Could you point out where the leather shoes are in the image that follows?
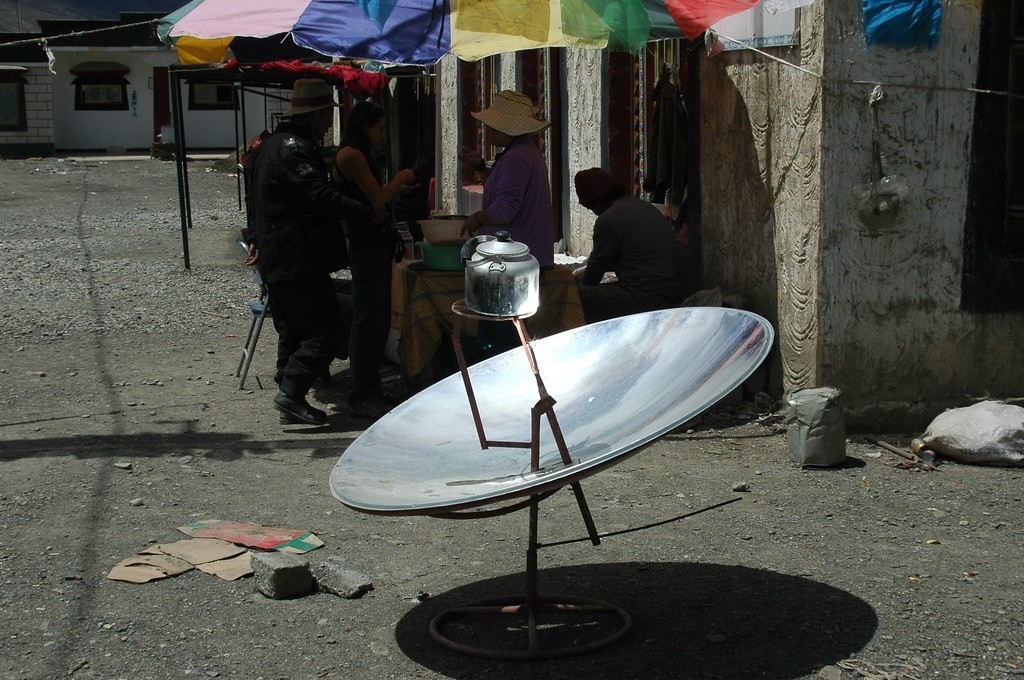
[272,390,328,425]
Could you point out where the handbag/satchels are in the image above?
[395,233,405,263]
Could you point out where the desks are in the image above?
[385,259,586,392]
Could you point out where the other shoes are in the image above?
[349,392,393,417]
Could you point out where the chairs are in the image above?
[234,229,272,391]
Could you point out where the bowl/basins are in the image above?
[417,220,479,242]
[416,242,465,271]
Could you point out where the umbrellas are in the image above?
[157,0,757,216]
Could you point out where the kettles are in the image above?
[462,231,540,320]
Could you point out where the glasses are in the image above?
[366,100,382,121]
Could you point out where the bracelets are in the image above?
[474,211,483,226]
[474,160,485,170]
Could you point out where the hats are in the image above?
[288,78,339,114]
[471,90,550,136]
[575,168,619,205]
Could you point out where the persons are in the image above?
[245,79,364,424]
[575,168,691,321]
[460,89,554,362]
[333,101,415,413]
